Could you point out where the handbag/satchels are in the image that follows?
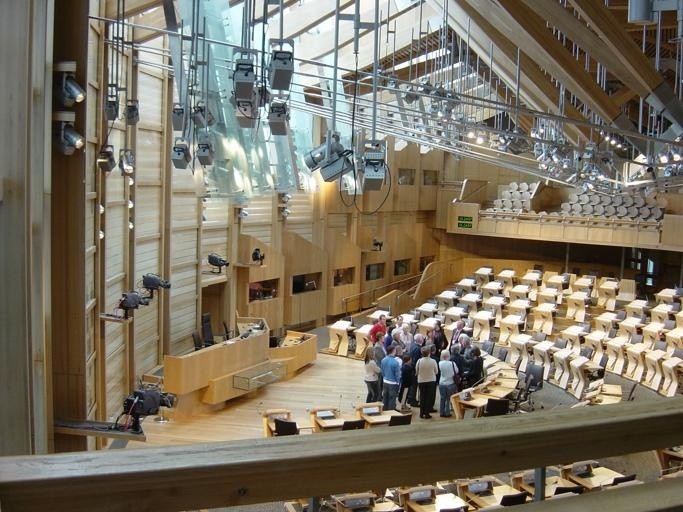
[453,373,462,385]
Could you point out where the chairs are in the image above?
[188,311,232,352]
[327,263,683,418]
[263,402,681,511]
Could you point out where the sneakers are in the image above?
[400,399,452,417]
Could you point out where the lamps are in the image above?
[529,84,683,195]
[227,0,300,137]
[50,58,143,179]
[303,2,397,217]
[386,0,528,158]
[167,0,221,173]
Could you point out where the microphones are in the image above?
[351,396,360,409]
[256,402,267,417]
[470,353,503,394]
[338,394,342,412]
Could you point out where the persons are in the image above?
[364,314,485,419]
